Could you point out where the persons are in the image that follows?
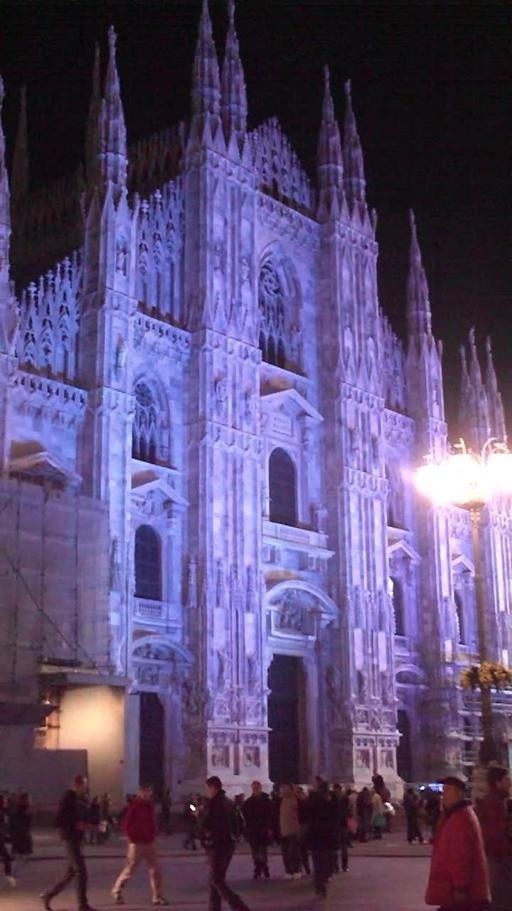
[199,776,251,911]
[242,769,395,899]
[40,772,101,911]
[0,789,42,887]
[235,793,245,842]
[84,788,172,847]
[424,762,512,911]
[181,793,211,853]
[110,775,169,905]
[403,786,444,845]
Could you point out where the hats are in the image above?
[436,776,469,791]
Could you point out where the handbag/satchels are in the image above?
[346,817,358,833]
[99,820,107,833]
[372,816,386,828]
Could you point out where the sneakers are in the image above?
[252,867,350,880]
[78,904,97,911]
[152,896,169,905]
[110,892,124,903]
[40,893,52,911]
